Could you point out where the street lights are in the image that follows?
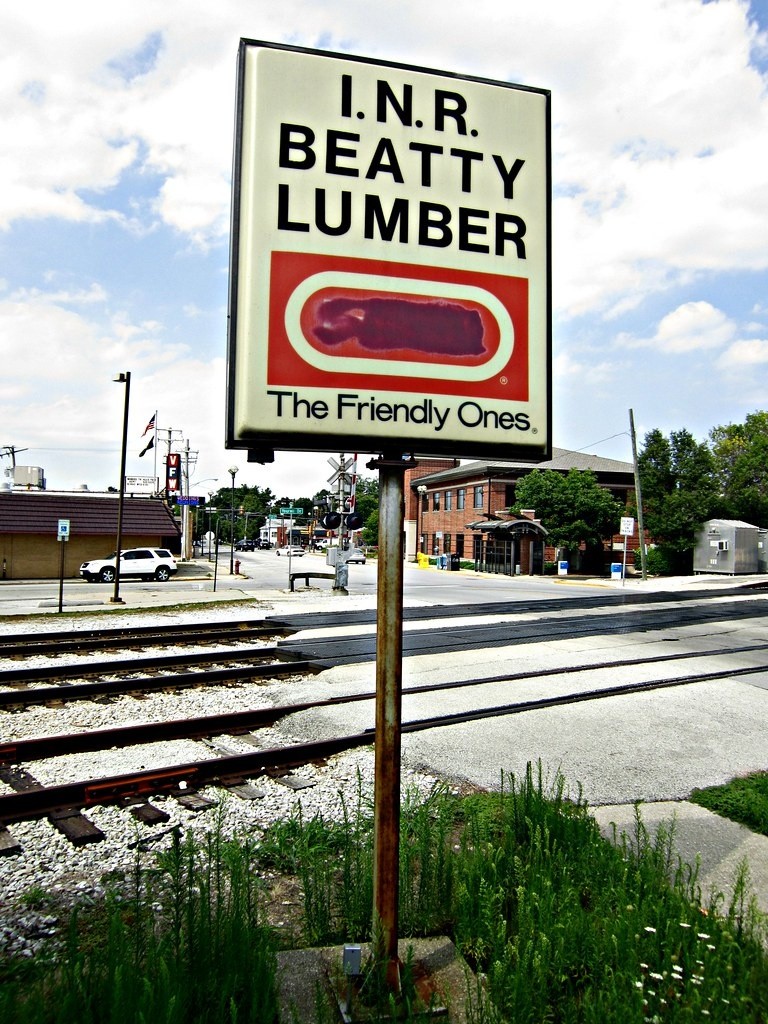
[227,466,238,575]
[112,369,132,600]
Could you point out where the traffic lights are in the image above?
[326,512,343,529]
[346,512,365,532]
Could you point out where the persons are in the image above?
[127,552,136,559]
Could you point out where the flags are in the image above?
[139,436,154,457]
[140,415,155,437]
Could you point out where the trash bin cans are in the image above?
[417,551,430,569]
[447,552,461,571]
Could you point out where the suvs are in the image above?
[78,545,178,582]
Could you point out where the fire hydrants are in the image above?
[235,559,241,575]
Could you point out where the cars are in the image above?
[276,545,306,558]
[345,548,366,565]
[314,537,344,550]
[233,538,273,551]
